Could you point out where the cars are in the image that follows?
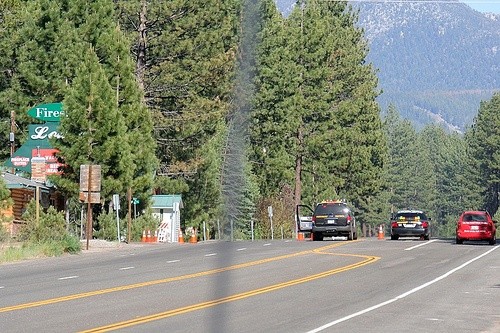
[453,211,498,244]
[389,209,431,241]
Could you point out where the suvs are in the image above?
[297,202,357,241]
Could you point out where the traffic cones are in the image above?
[141,231,157,243]
[376,224,384,239]
[178,231,184,244]
[189,231,198,244]
[297,233,304,239]
